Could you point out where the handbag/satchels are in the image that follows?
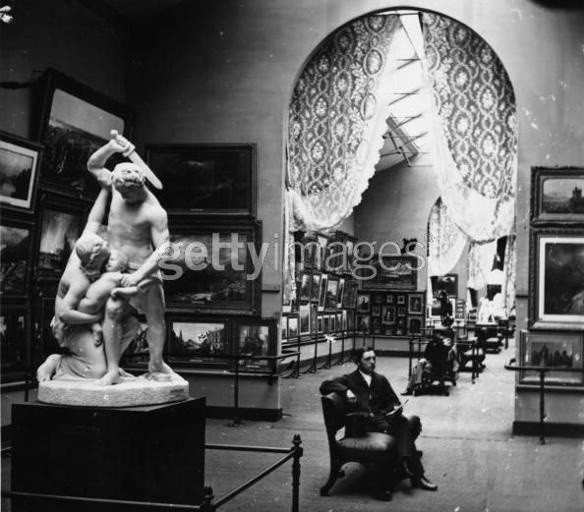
[346,413,370,438]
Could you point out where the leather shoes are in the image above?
[412,476,437,490]
[398,461,414,477]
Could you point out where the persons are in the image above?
[36,167,139,382]
[87,134,175,386]
[321,346,438,491]
[77,251,139,346]
[401,329,453,395]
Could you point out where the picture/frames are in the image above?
[0,67,277,394]
[282,230,426,348]
[528,229,584,329]
[529,166,584,227]
[519,330,584,386]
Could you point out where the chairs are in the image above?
[457,336,485,376]
[423,350,456,395]
[320,394,398,501]
[477,327,503,353]
